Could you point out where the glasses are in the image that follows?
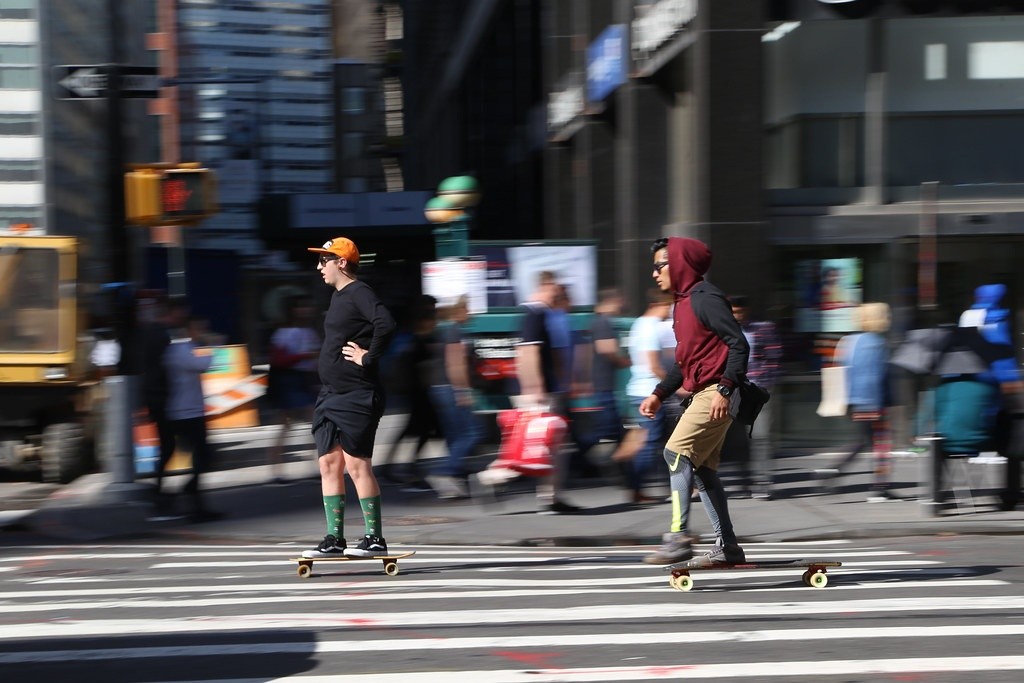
[318,255,341,265]
[650,261,669,272]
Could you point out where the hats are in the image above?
[307,237,360,264]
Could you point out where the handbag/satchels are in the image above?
[491,410,567,474]
[728,381,770,425]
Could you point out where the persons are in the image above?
[147,271,1024,525]
[638,237,750,567]
[300,236,395,559]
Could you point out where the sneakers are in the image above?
[688,544,746,565]
[344,533,388,557]
[644,537,694,563]
[302,534,347,557]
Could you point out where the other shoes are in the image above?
[538,502,579,515]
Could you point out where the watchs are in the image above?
[717,385,733,397]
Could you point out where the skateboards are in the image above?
[663,561,842,592]
[289,550,417,578]
